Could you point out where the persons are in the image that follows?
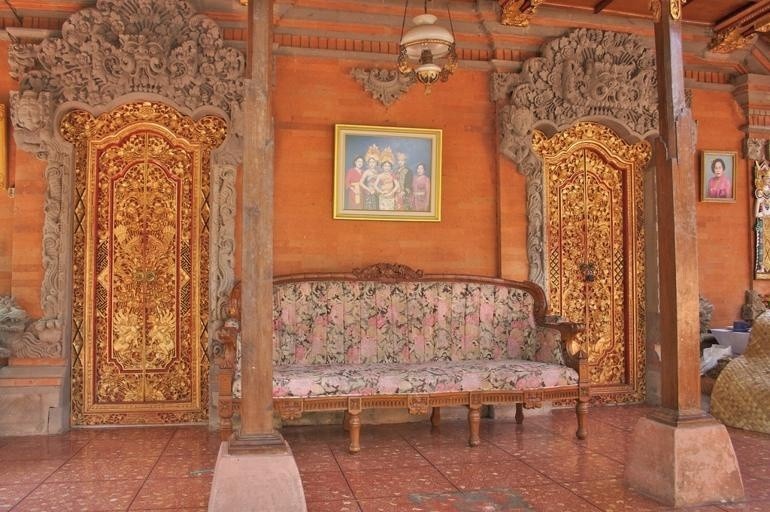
[345,153,431,211]
[709,158,731,198]
[754,168,770,274]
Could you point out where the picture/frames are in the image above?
[332,124,442,222]
[700,150,736,202]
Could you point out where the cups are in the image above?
[733,319,748,329]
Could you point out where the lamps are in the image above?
[397,0,458,96]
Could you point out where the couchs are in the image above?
[217,262,591,453]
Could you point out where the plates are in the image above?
[732,327,749,332]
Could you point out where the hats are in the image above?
[397,152,406,161]
[364,144,380,162]
[380,146,393,164]
